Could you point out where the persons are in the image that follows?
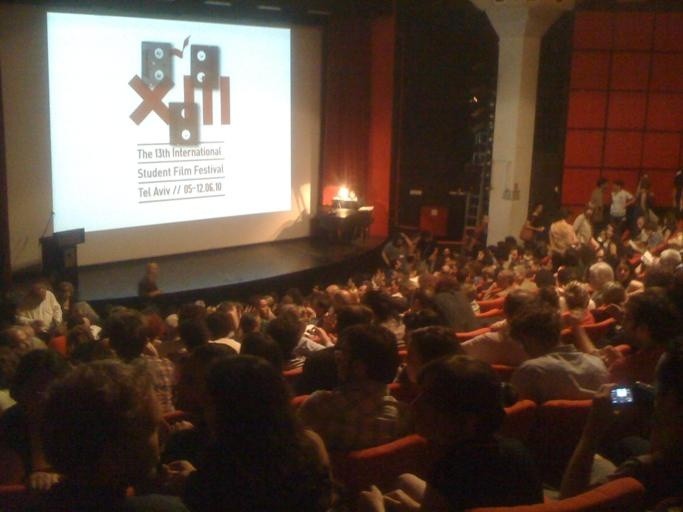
[632,172,655,222]
[585,176,606,226]
[1,279,683,511]
[359,200,682,277]
[607,178,637,222]
[137,261,161,309]
[671,167,681,224]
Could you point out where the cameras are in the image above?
[610,386,635,407]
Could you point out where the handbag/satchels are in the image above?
[520,224,534,241]
